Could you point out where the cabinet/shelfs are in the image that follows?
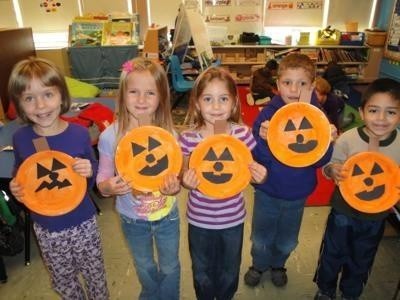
[1,26,37,118]
[211,44,370,80]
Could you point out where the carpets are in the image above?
[235,85,338,208]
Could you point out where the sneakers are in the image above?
[246,93,255,106]
[255,97,272,106]
[314,289,336,300]
[271,267,287,286]
[244,266,262,287]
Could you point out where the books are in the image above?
[211,48,367,64]
[71,11,141,46]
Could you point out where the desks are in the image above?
[0,98,117,266]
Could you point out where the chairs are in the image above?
[169,55,198,111]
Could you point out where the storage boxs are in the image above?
[38,47,69,79]
[72,43,140,90]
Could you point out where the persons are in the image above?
[10,56,111,300]
[94,57,182,300]
[177,66,269,300]
[243,52,339,289]
[312,76,400,300]
[245,59,276,106]
[321,62,351,102]
[314,78,345,121]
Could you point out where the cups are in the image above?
[285,35,292,45]
[296,32,310,45]
